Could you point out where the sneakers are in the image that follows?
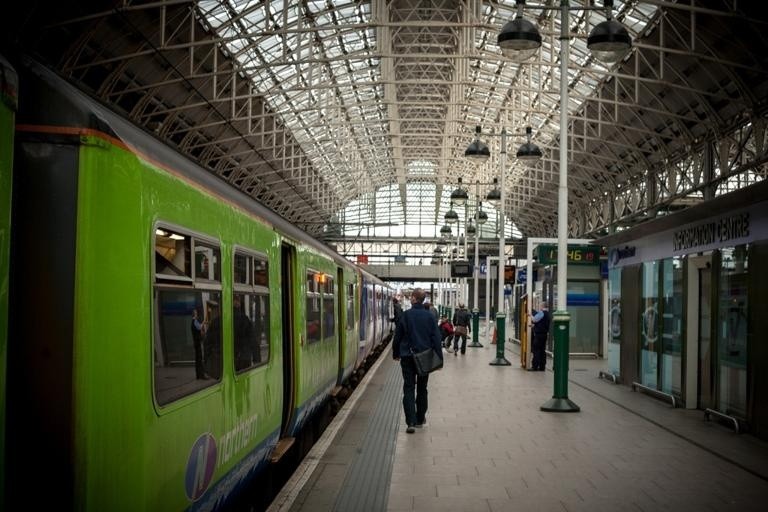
[455,349,458,355]
[416,418,426,427]
[407,422,415,432]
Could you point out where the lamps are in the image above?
[430,125,542,266]
[496,0,632,63]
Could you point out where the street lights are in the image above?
[463,124,544,368]
[430,177,500,347]
[497,0,632,413]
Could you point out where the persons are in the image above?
[424,300,472,356]
[190,296,262,382]
[392,287,443,434]
[388,298,404,328]
[527,301,551,372]
[308,302,333,338]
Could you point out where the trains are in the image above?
[0,59,406,510]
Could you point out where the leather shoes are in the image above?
[527,367,545,371]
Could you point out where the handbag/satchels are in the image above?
[413,348,442,376]
[455,326,467,336]
